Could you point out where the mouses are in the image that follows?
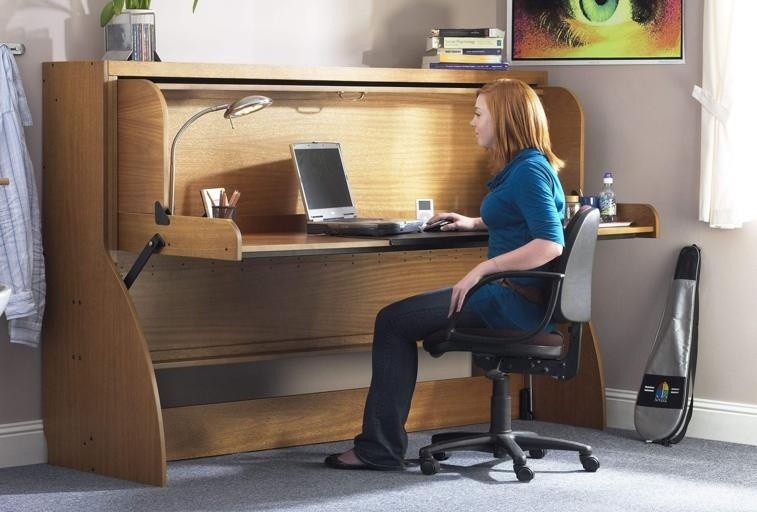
[424,220,455,234]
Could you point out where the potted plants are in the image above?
[99,0,161,61]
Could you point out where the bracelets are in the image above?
[490,257,502,272]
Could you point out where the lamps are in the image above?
[168,94,274,216]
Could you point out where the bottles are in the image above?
[566,172,618,222]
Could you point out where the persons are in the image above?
[324,78,566,471]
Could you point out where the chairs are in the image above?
[418,208,600,483]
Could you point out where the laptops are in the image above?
[287,140,423,238]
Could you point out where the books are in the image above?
[104,9,156,62]
[420,28,509,71]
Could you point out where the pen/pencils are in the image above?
[206,188,241,219]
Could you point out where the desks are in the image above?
[35,61,662,487]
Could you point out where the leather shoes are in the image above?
[325,454,379,470]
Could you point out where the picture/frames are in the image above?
[506,1,686,66]
